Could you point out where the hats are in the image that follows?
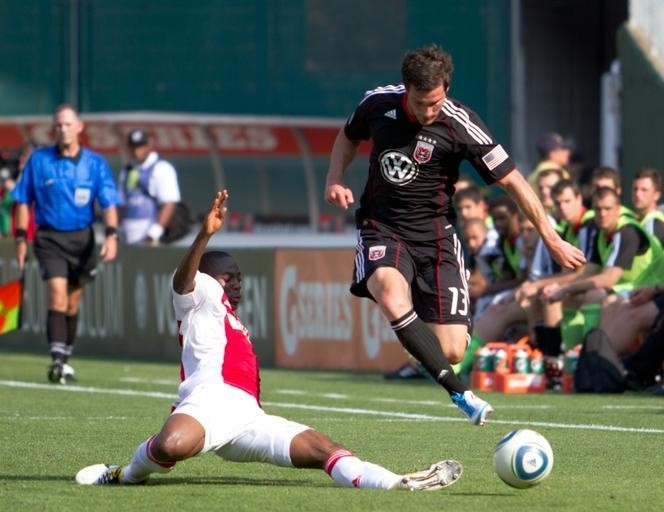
[536,133,573,158]
[128,129,150,147]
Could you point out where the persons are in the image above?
[527,187,664,392]
[591,167,641,222]
[538,170,568,235]
[469,196,529,299]
[1,142,37,333]
[76,188,462,492]
[461,219,500,284]
[454,187,495,232]
[10,104,119,384]
[526,133,574,202]
[116,129,181,365]
[448,181,601,385]
[521,215,543,282]
[323,48,586,425]
[576,284,664,393]
[631,170,663,250]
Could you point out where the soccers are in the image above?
[493,429,554,488]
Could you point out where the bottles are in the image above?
[529,350,543,376]
[512,348,529,375]
[474,345,496,372]
[564,344,583,377]
[492,348,508,372]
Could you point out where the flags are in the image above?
[0,280,20,336]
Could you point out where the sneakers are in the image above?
[449,389,493,427]
[397,458,461,491]
[75,464,149,486]
[384,364,422,380]
[49,367,65,384]
[62,364,74,379]
